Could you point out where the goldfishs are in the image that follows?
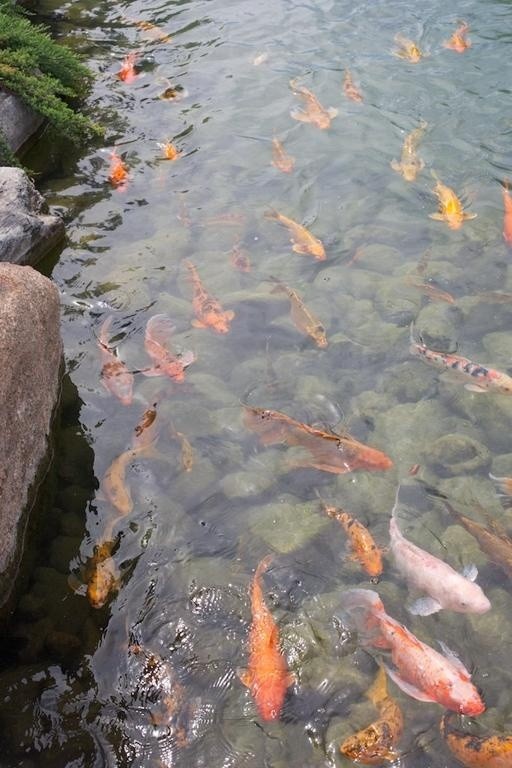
[60,6,512,768]
